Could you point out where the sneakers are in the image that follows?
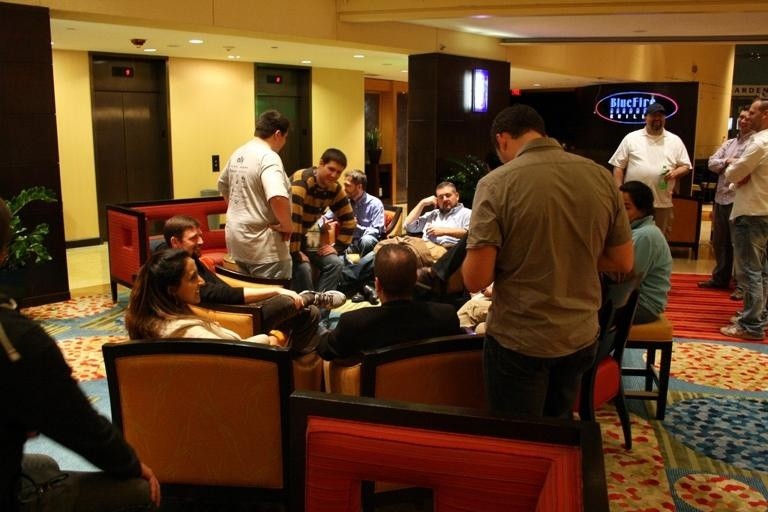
[721,311,766,340]
[698,277,731,289]
[297,289,347,310]
[729,288,746,299]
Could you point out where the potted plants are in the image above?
[366,128,383,163]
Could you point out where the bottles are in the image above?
[659,166,668,191]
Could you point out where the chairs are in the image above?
[215,265,291,293]
[572,288,641,449]
[665,194,703,259]
[185,303,261,341]
[101,339,292,512]
[323,334,490,413]
[384,206,403,239]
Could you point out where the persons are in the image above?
[698,100,768,340]
[608,104,693,234]
[0,199,167,512]
[462,103,635,419]
[218,110,386,290]
[403,181,472,263]
[318,243,460,365]
[124,215,345,355]
[618,180,671,323]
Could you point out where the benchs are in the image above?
[104,196,227,303]
[283,387,609,512]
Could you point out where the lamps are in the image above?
[472,67,489,114]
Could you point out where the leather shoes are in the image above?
[352,283,378,303]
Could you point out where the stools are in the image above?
[619,314,674,420]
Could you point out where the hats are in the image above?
[645,104,665,114]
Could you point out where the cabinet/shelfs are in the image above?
[365,164,393,206]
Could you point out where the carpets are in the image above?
[20,281,132,472]
[594,275,768,512]
